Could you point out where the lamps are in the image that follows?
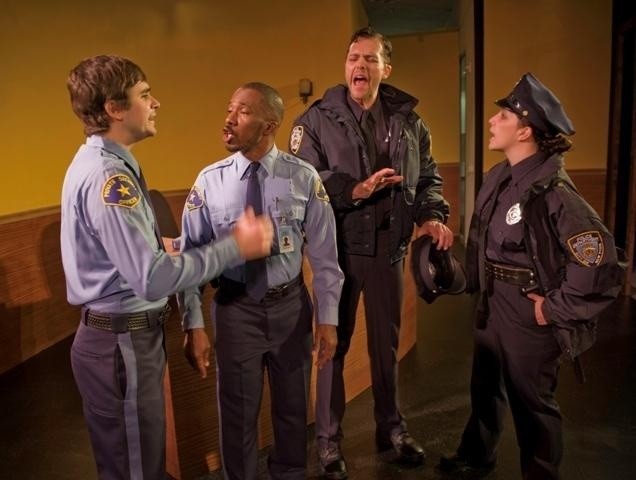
[297,78,313,105]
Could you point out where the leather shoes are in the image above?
[316,445,348,480]
[377,430,426,464]
[441,452,497,479]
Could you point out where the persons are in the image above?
[430,74,630,480]
[173,81,345,480]
[292,24,456,475]
[57,54,274,480]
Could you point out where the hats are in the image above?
[494,73,575,135]
[411,234,468,305]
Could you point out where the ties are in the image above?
[360,111,377,168]
[137,166,166,252]
[245,162,271,304]
[479,171,513,292]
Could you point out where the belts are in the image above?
[86,304,171,332]
[263,278,301,303]
[483,261,536,288]
